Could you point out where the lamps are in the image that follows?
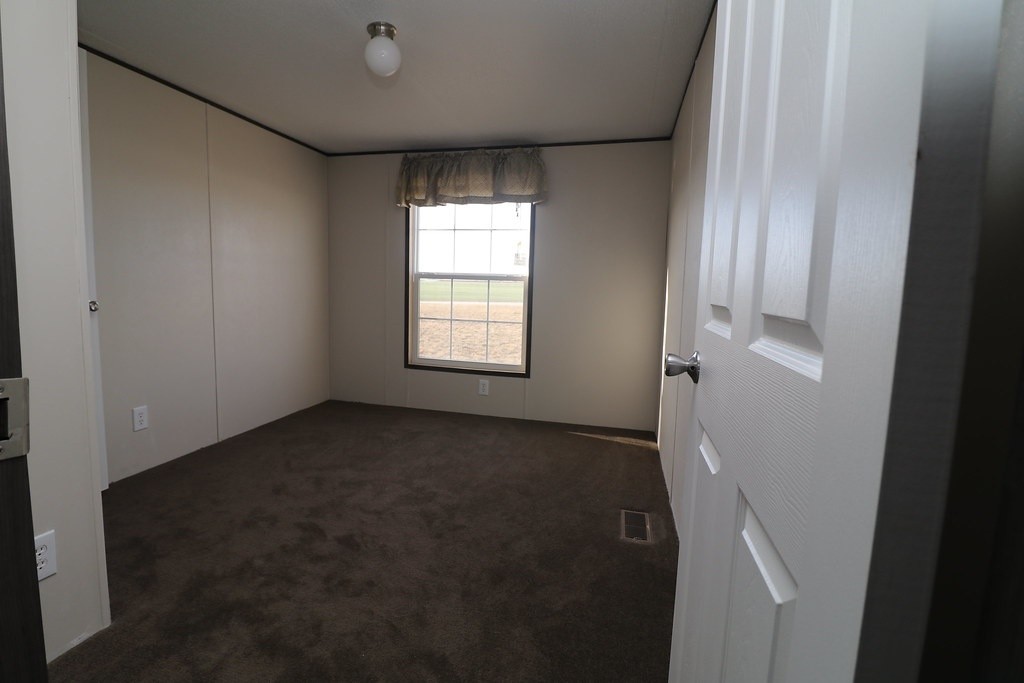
[365,21,401,77]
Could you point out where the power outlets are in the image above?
[133,406,148,432]
[34,529,56,582]
[478,379,489,396]
